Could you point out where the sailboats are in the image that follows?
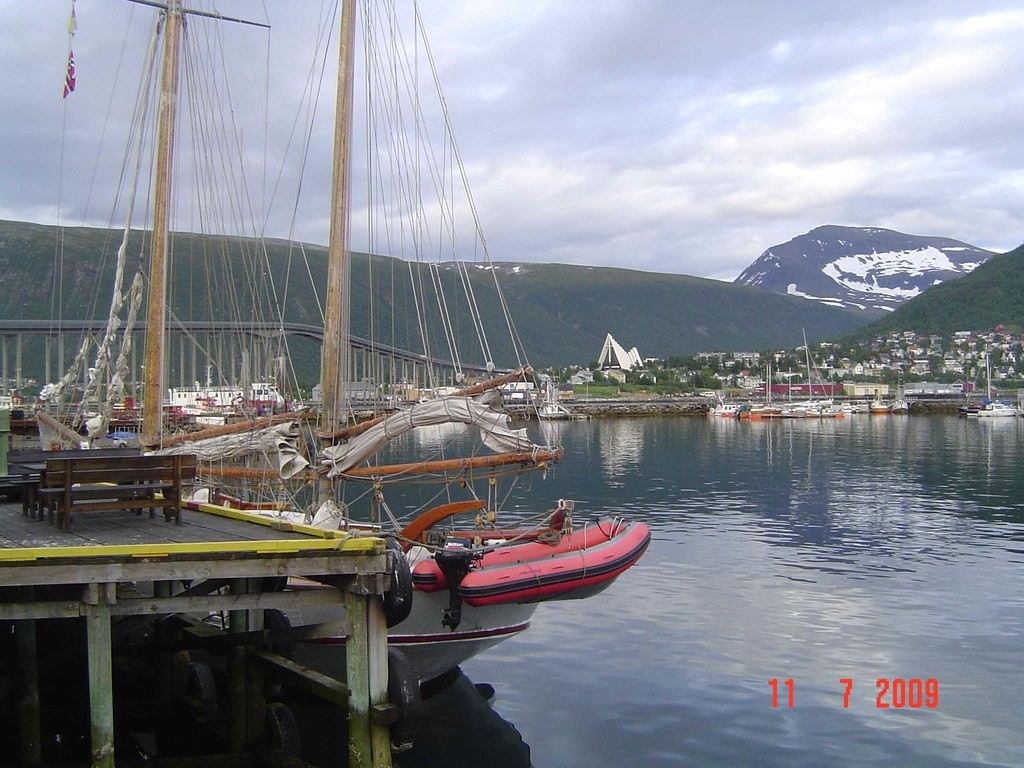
[956,351,1018,417]
[36,2,651,705]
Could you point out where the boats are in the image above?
[870,385,909,414]
[704,327,869,418]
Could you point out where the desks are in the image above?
[19,462,155,518]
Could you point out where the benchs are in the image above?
[7,447,142,521]
[38,454,198,531]
